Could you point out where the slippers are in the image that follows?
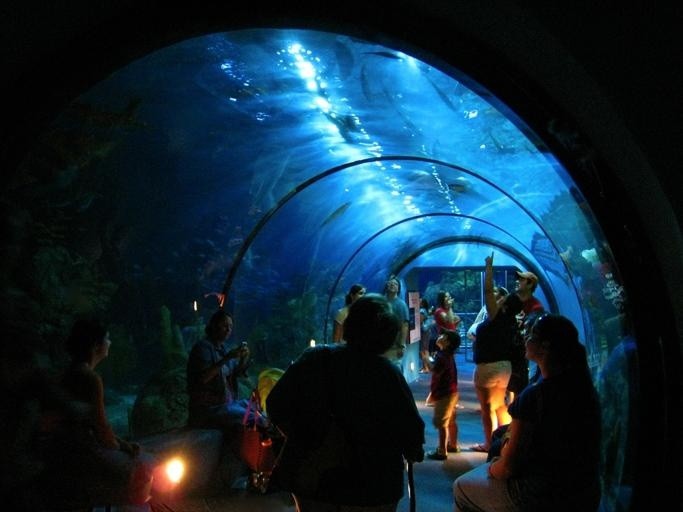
[447,441,458,452]
[428,447,446,460]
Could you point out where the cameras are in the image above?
[242,341,248,349]
[429,356,436,363]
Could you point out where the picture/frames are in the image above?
[406,290,422,345]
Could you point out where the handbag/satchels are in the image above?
[238,427,274,472]
[130,376,191,439]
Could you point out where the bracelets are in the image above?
[489,466,495,479]
[399,344,406,348]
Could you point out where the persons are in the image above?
[452,312,602,512]
[419,289,461,460]
[186,311,268,496]
[381,275,410,363]
[468,251,544,454]
[333,284,366,345]
[39,320,156,512]
[266,298,425,512]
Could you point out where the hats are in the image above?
[515,271,538,283]
[440,326,460,349]
